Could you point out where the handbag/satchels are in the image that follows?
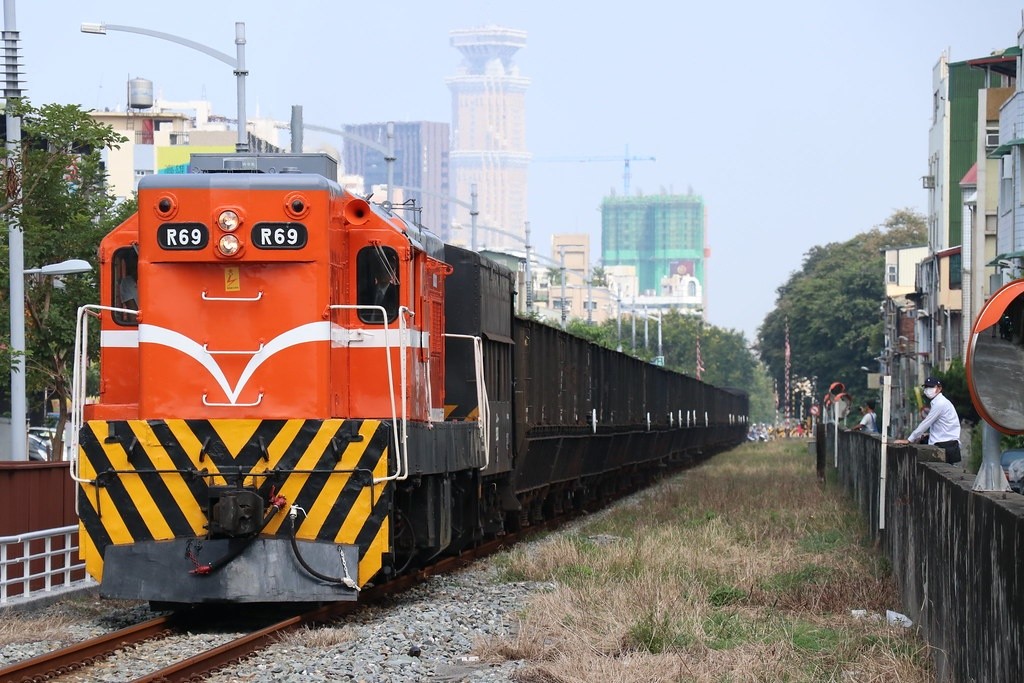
[934,439,962,463]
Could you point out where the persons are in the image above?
[854,399,878,432]
[749,421,810,442]
[893,378,961,463]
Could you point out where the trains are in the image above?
[69,153,752,637]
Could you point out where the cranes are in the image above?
[578,146,656,197]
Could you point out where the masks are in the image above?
[924,386,940,399]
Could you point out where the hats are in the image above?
[922,376,942,387]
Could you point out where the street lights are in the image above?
[81,13,248,157]
